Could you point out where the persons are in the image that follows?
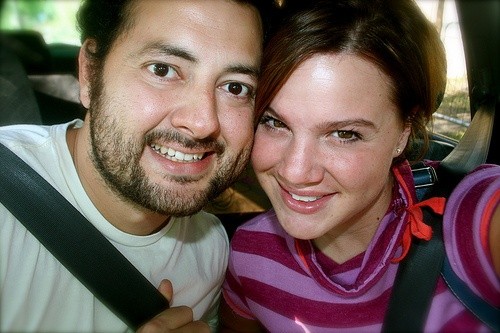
[0,0,281,333]
[221,0,500,333]
